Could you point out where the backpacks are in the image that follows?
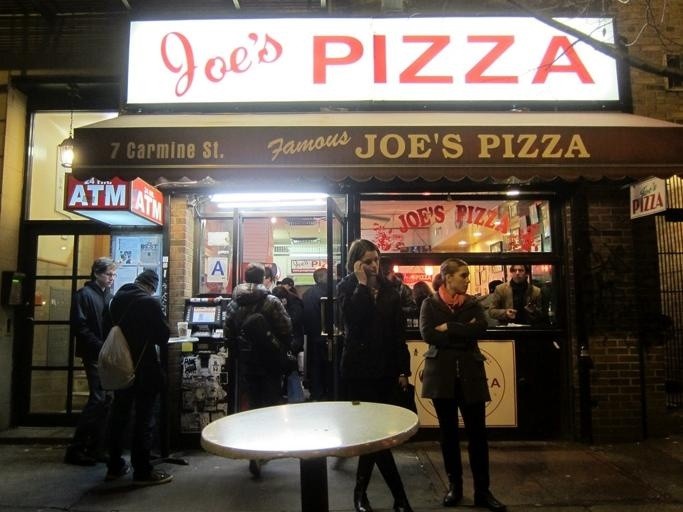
[97,297,154,389]
[237,294,299,379]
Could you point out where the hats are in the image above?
[134,269,158,292]
[277,278,294,285]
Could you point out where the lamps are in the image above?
[56,111,75,168]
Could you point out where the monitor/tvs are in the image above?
[185,303,222,338]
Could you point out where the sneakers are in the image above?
[64,450,95,466]
[132,470,174,484]
[85,446,109,463]
[105,462,130,479]
[473,488,506,511]
[443,487,462,506]
[248,459,261,476]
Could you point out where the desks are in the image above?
[198,400,420,512]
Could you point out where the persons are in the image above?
[105,268,172,485]
[64,257,117,465]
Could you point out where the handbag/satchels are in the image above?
[382,382,417,414]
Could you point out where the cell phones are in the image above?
[361,264,369,273]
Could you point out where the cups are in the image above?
[177,322,188,339]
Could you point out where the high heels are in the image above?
[354,487,372,512]
[393,499,414,512]
